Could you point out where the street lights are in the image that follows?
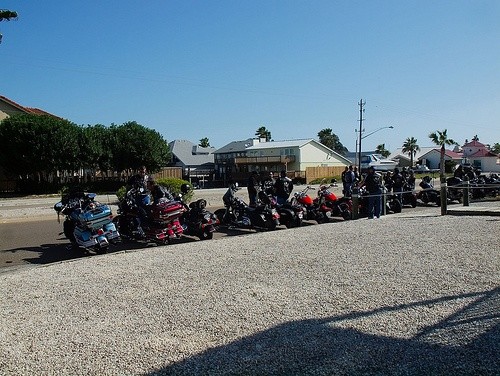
[361,125,394,140]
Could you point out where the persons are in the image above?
[243,162,489,213]
[128,166,156,208]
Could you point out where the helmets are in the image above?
[181,183,194,194]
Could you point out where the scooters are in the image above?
[214,182,306,232]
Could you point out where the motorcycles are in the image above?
[290,184,333,225]
[113,186,188,246]
[172,184,220,240]
[312,178,352,221]
[53,188,121,255]
[386,168,500,214]
